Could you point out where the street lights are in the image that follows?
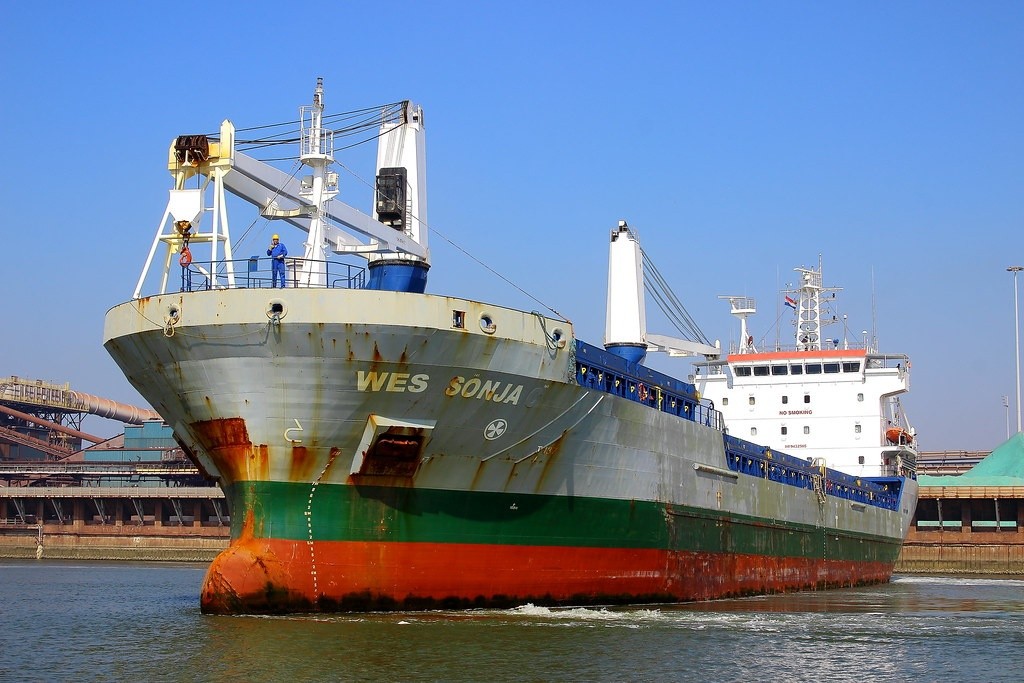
[1006,265,1024,431]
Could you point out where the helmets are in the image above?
[273,234,279,240]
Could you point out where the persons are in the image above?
[267,235,288,288]
[894,464,898,475]
[7,424,10,429]
[27,431,31,436]
[897,364,900,368]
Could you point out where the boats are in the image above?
[101,75,920,619]
[886,426,914,445]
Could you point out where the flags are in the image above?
[785,295,797,310]
